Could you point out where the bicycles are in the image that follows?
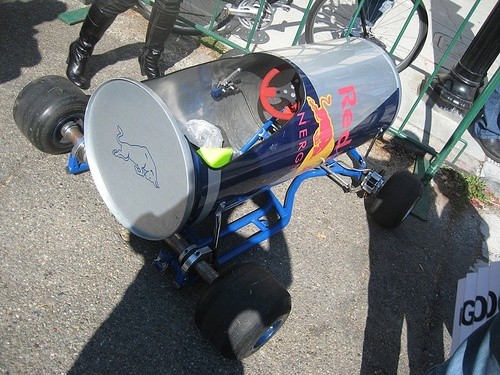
[131,0,428,73]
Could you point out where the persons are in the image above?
[471,85,500,165]
[66,0,183,90]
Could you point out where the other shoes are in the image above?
[350,32,386,50]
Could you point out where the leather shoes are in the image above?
[477,138,500,162]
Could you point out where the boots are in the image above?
[65,2,114,89]
[139,4,179,80]
